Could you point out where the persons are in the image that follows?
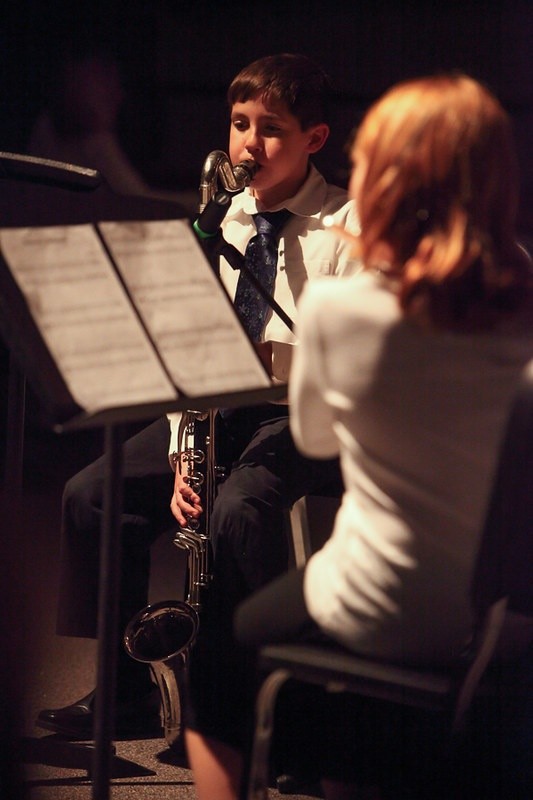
[10,54,199,213]
[36,53,350,735]
[179,73,533,800]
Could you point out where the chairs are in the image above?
[246,358,533,800]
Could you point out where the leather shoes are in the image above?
[38,679,162,735]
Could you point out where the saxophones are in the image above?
[124,150,264,748]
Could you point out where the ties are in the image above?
[231,211,287,349]
